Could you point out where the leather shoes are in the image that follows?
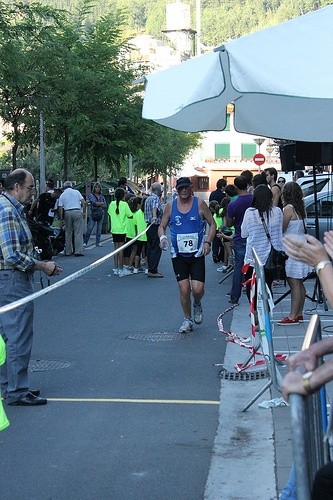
[25,388,40,396]
[8,392,47,406]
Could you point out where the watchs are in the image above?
[316,260,331,275]
[302,370,316,395]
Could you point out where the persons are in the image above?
[0,168,64,405]
[294,170,304,181]
[83,182,106,247]
[157,177,217,332]
[108,182,164,278]
[276,181,315,325]
[28,178,88,261]
[281,337,333,499]
[282,231,333,312]
[208,167,286,303]
[240,184,283,331]
[308,166,323,175]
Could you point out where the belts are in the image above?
[65,208,80,211]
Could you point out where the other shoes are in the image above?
[66,254,71,256]
[146,272,164,278]
[229,300,233,304]
[82,243,86,246]
[75,254,84,256]
[98,243,103,247]
[225,292,231,298]
[113,258,148,276]
[217,266,228,273]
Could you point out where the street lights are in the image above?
[253,137,266,173]
[266,148,273,167]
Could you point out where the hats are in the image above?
[175,177,193,189]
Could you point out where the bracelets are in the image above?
[205,241,211,247]
[160,235,167,241]
[29,210,33,211]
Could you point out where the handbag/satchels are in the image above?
[265,247,289,281]
[91,210,103,221]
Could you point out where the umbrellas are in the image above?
[133,5,333,142]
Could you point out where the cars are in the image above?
[274,171,333,245]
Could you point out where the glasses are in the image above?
[178,185,190,191]
[17,183,35,190]
[278,180,285,183]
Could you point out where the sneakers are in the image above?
[277,317,299,326]
[193,302,203,325]
[179,317,194,333]
[297,315,304,322]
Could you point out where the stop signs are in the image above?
[253,153,266,166]
